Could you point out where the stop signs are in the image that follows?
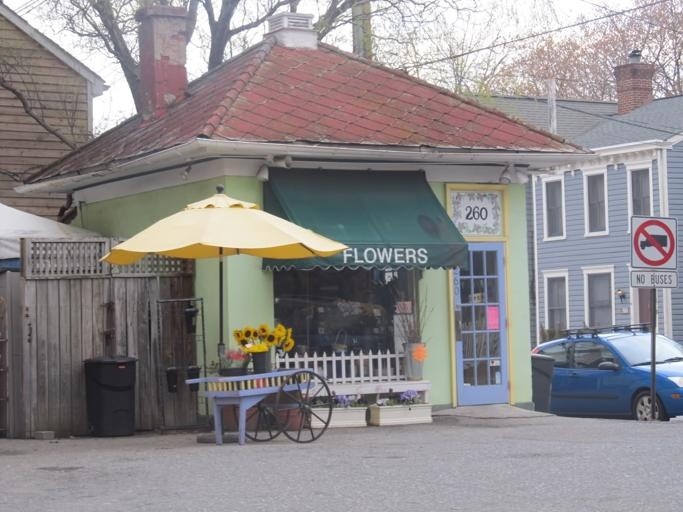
[629,214,679,289]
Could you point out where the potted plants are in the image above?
[391,281,437,381]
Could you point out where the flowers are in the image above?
[220,323,295,368]
[314,390,423,407]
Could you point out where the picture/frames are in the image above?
[446,183,509,242]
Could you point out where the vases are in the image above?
[219,350,272,376]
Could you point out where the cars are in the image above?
[528,323,683,421]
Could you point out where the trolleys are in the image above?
[186,367,334,445]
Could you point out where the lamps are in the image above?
[500,162,522,184]
[256,163,269,182]
[180,166,192,181]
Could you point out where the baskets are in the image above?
[331,343,347,352]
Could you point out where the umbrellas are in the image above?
[94,182,350,430]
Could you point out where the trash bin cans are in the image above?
[84,355,139,437]
[531,354,555,412]
